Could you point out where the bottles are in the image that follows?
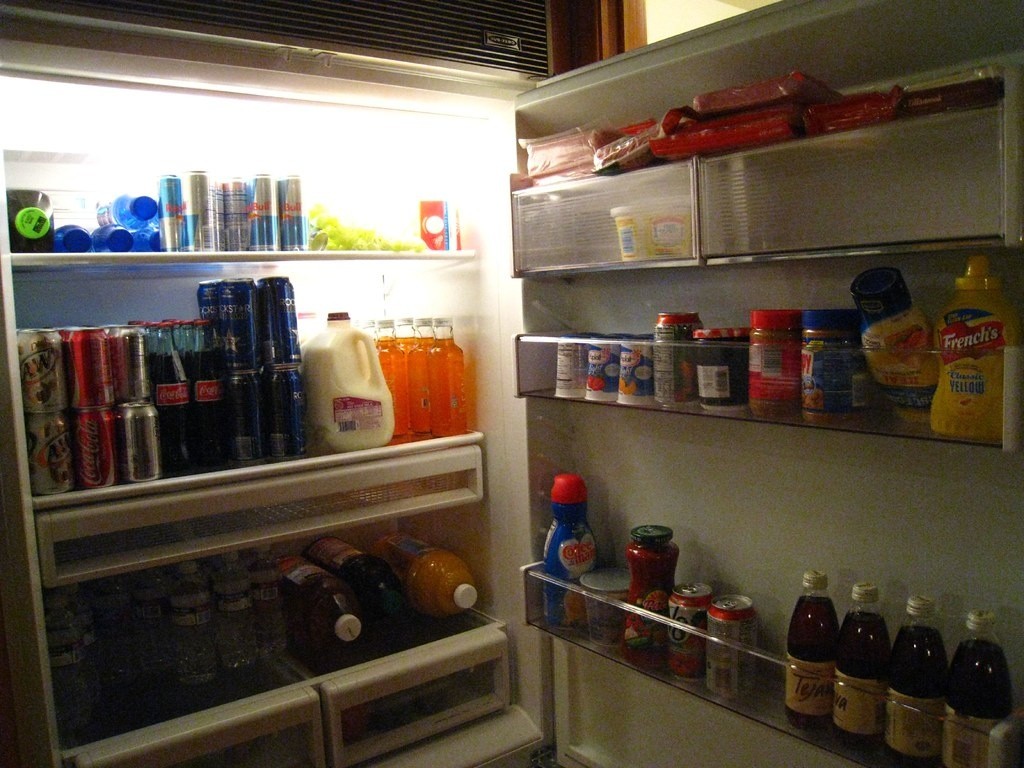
[41,550,286,731]
[883,594,948,768]
[784,569,840,732]
[297,310,467,453]
[941,607,1012,768]
[275,552,365,642]
[622,524,681,653]
[831,581,892,747]
[301,536,406,625]
[370,531,476,617]
[541,472,597,631]
[6,188,161,252]
[849,253,1021,444]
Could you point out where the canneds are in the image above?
[706,594,756,698]
[17,324,163,495]
[693,327,752,411]
[798,308,874,427]
[159,170,309,253]
[664,583,715,682]
[750,309,802,419]
[618,523,680,667]
[652,310,704,407]
[196,276,308,462]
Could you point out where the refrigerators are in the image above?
[0,0,1024,768]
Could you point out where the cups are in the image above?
[578,567,631,648]
